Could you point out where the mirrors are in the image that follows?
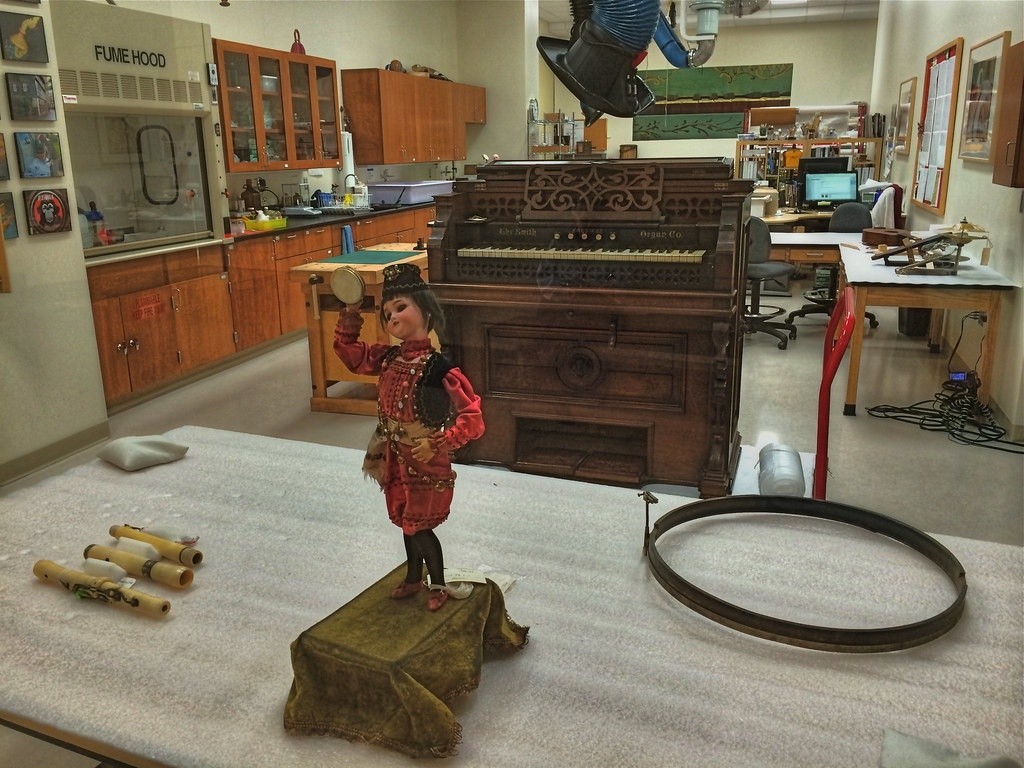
[958,30,1011,164]
[894,77,916,155]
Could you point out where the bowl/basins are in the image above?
[738,134,754,141]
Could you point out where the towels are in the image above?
[341,224,354,255]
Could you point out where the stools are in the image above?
[283,560,527,758]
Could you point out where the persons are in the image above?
[333,263,485,611]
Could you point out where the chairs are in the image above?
[812,183,903,295]
[745,215,797,350]
[784,202,879,346]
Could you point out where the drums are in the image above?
[330,265,366,305]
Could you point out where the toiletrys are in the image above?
[366,168,375,182]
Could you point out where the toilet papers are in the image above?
[308,169,324,175]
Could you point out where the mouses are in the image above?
[794,209,801,213]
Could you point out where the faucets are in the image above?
[380,168,395,182]
[441,166,458,177]
[343,174,361,194]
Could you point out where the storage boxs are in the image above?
[245,217,287,230]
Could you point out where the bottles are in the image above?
[294,193,303,207]
[248,208,255,215]
[264,207,268,216]
[767,126,782,140]
[282,192,292,207]
[274,208,281,219]
[241,178,261,212]
[874,188,882,205]
[235,195,245,212]
[808,129,815,140]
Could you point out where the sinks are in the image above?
[366,180,456,204]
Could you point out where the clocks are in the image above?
[530,98,537,121]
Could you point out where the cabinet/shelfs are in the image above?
[86,203,436,399]
[527,109,574,160]
[992,41,1024,188]
[341,68,486,164]
[213,38,343,173]
[735,138,882,206]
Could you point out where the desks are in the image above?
[290,242,429,416]
[747,208,906,297]
[0,426,1024,768]
[769,232,1018,416]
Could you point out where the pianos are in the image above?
[426,155,757,500]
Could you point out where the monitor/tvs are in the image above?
[797,157,849,204]
[803,171,858,210]
